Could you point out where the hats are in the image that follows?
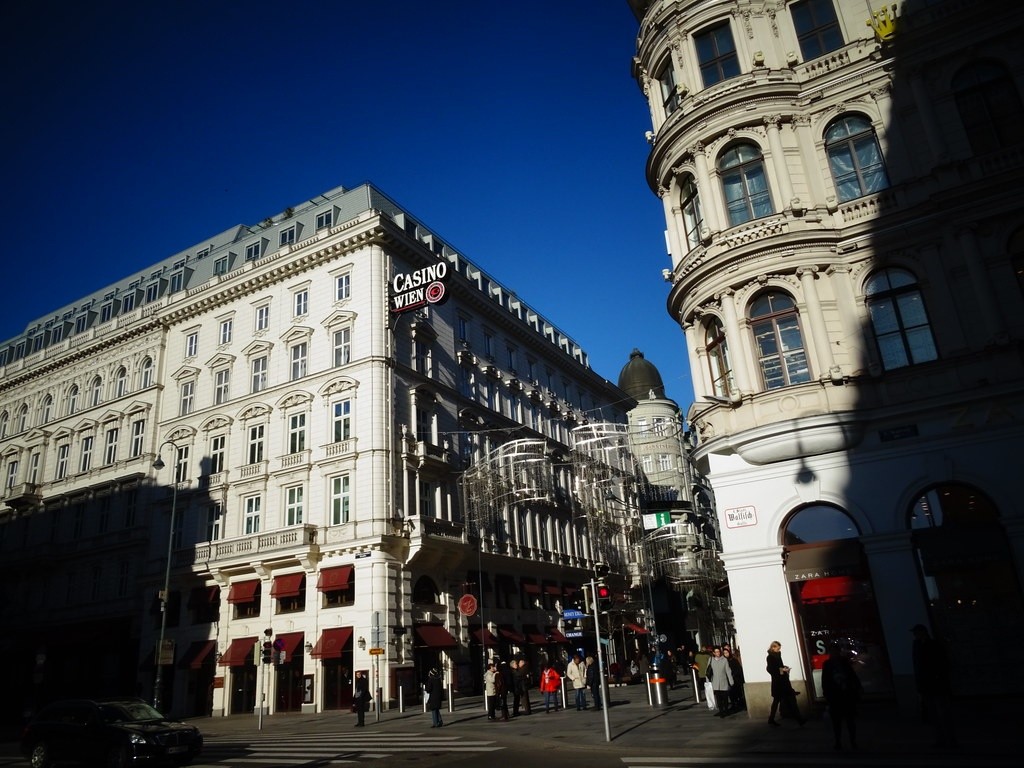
[908,624,928,633]
[431,668,438,676]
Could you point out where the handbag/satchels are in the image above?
[495,698,503,710]
[706,657,713,682]
[774,675,792,696]
[704,682,717,710]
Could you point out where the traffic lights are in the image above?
[594,563,612,613]
[264,629,273,636]
[263,642,272,664]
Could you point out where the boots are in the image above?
[591,704,601,711]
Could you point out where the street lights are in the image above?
[606,489,662,672]
[151,441,182,708]
[475,491,554,711]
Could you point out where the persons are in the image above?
[425,668,444,728]
[822,647,864,752]
[695,642,743,711]
[627,645,688,690]
[766,641,808,727]
[539,662,561,713]
[585,657,601,711]
[567,655,589,711]
[483,659,532,721]
[352,671,372,727]
[909,624,961,749]
[705,645,734,718]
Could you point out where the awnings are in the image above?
[309,627,353,659]
[271,631,304,663]
[187,563,354,611]
[415,625,458,650]
[624,623,651,634]
[178,640,216,669]
[218,637,258,666]
[470,624,569,646]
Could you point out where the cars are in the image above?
[22,695,205,768]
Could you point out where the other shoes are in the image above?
[355,723,363,726]
[577,706,581,711]
[488,713,530,720]
[432,721,442,728]
[798,718,808,726]
[583,707,588,710]
[769,719,781,726]
